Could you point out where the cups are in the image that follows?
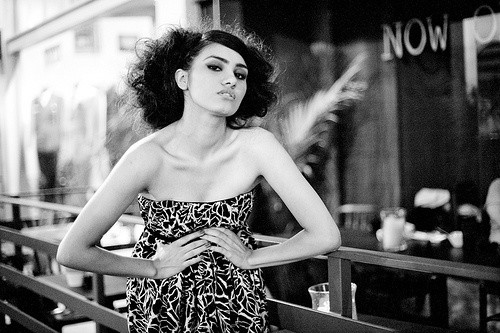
[308,281,359,320]
[381,208,406,252]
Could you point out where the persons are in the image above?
[55,18,341,331]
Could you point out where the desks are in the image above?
[339,228,449,328]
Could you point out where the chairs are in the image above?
[333,204,380,233]
